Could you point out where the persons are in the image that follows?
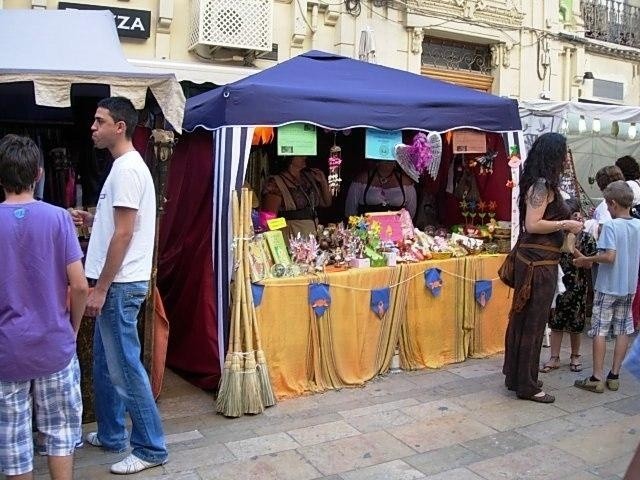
[265,157,332,240]
[587,166,625,341]
[499,132,586,404]
[540,197,597,372]
[615,155,640,334]
[71,96,170,475]
[0,133,88,479]
[346,155,418,220]
[573,181,640,394]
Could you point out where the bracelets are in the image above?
[552,220,567,232]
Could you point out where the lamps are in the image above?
[594,117,600,130]
[579,116,587,134]
[630,125,636,137]
[612,122,618,137]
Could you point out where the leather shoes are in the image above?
[537,376,544,388]
[519,391,555,403]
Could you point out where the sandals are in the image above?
[540,356,562,373]
[568,353,582,372]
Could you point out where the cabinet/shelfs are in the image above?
[243,249,516,397]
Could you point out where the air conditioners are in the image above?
[186,0,273,65]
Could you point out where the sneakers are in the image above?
[574,375,605,393]
[604,375,620,391]
[110,451,169,475]
[85,431,104,446]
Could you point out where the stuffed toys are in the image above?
[253,210,260,230]
[459,201,469,225]
[328,132,343,197]
[256,235,272,273]
[272,244,290,278]
[264,231,288,264]
[268,217,287,230]
[507,144,522,188]
[263,211,275,229]
[395,132,443,184]
[468,201,477,225]
[477,199,487,226]
[488,200,497,222]
[276,207,484,276]
[467,150,498,176]
[249,239,267,282]
